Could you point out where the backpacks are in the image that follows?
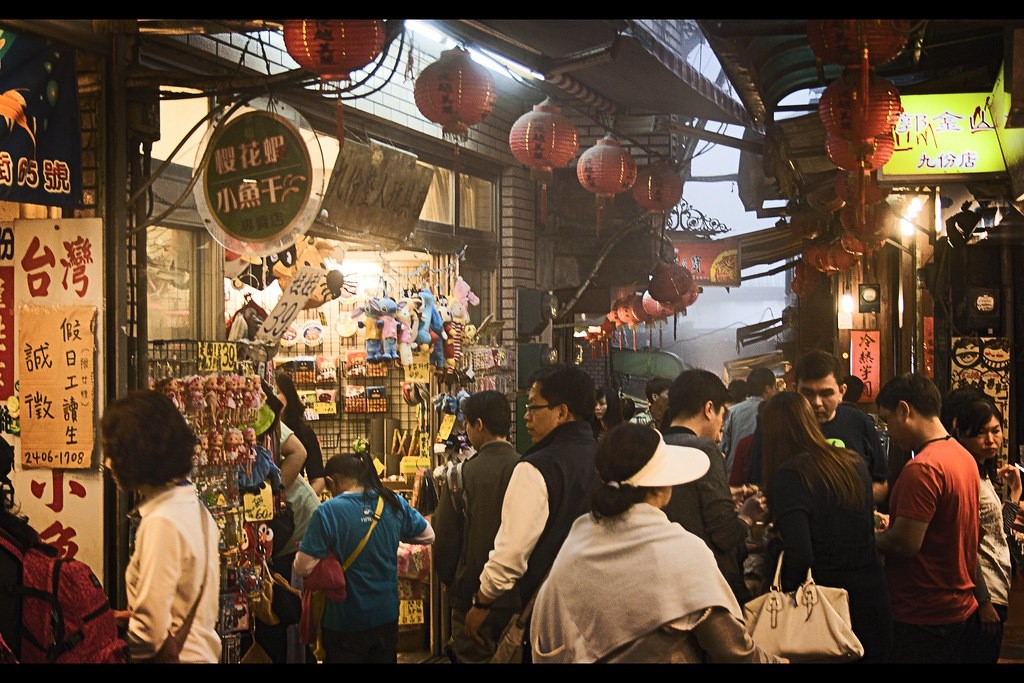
[0,528,130,666]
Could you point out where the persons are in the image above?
[530,424,790,663]
[875,372,982,663]
[0,476,129,664]
[100,390,222,663]
[293,451,435,663]
[761,392,892,664]
[266,373,326,663]
[434,390,523,664]
[952,399,1022,664]
[464,351,889,663]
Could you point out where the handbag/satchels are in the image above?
[742,549,866,664]
[308,587,328,660]
[255,548,304,625]
[150,629,180,662]
[488,611,526,663]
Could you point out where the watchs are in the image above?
[472,592,496,610]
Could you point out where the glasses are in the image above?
[720,401,731,423]
[525,403,550,413]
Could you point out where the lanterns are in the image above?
[583,261,704,345]
[414,50,497,173]
[833,171,892,205]
[817,70,904,171]
[283,18,385,149]
[577,139,683,238]
[806,20,911,121]
[791,200,901,297]
[509,105,579,226]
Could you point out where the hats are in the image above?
[596,426,711,487]
